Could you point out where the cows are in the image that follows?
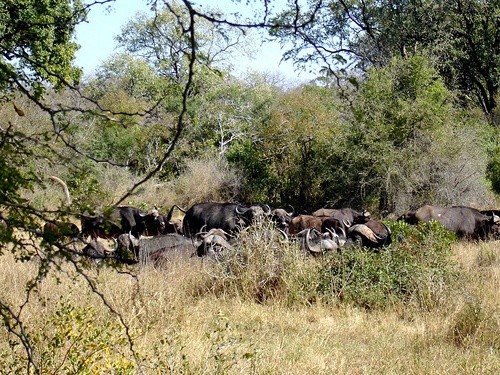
[42,201,500,261]
